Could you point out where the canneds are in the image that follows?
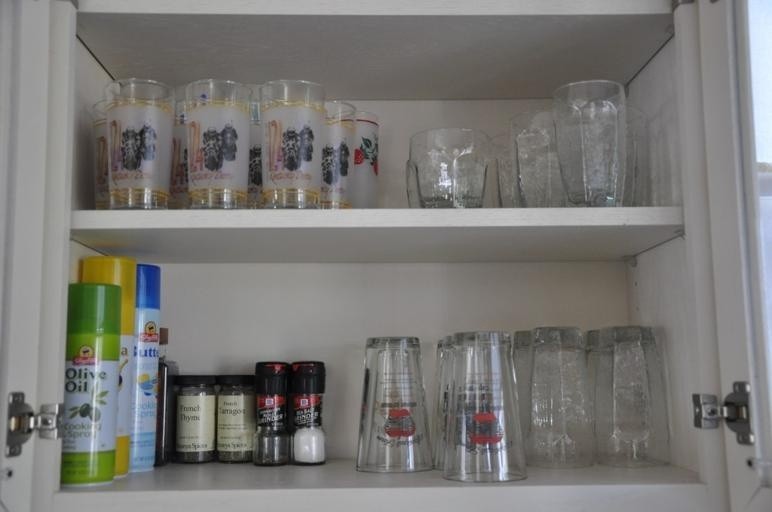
[172,375,216,463]
[216,375,256,464]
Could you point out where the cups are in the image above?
[407,127,491,208]
[495,79,643,209]
[352,335,438,474]
[432,324,671,482]
[87,78,382,210]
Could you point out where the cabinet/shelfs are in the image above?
[54,2,725,511]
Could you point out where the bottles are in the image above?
[155,326,171,464]
[61,282,121,492]
[173,360,326,466]
[129,264,160,475]
[83,254,135,478]
